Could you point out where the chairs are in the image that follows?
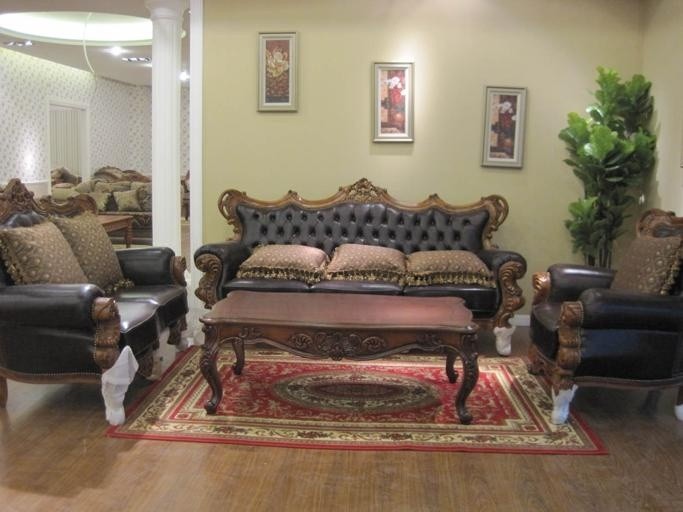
[51,167,83,206]
[528,209,683,424]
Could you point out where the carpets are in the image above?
[104,343,610,456]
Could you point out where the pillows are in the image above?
[0,218,88,285]
[609,236,683,296]
[236,244,331,285]
[48,208,134,291]
[51,183,75,201]
[75,178,152,212]
[404,245,498,288]
[324,242,406,283]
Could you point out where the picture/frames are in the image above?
[256,30,299,113]
[480,85,527,168]
[372,59,414,143]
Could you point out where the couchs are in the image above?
[83,165,152,244]
[0,177,188,428]
[193,177,527,357]
